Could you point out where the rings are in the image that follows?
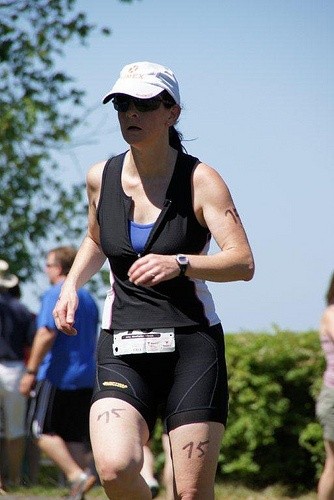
[147,270,155,278]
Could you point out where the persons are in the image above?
[315,270,334,500]
[0,244,177,500]
[52,59,255,500]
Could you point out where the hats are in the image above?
[0,260,18,288]
[103,61,180,105]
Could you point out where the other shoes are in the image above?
[67,471,96,500]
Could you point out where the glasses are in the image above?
[112,93,173,112]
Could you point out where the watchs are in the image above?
[176,253,189,277]
